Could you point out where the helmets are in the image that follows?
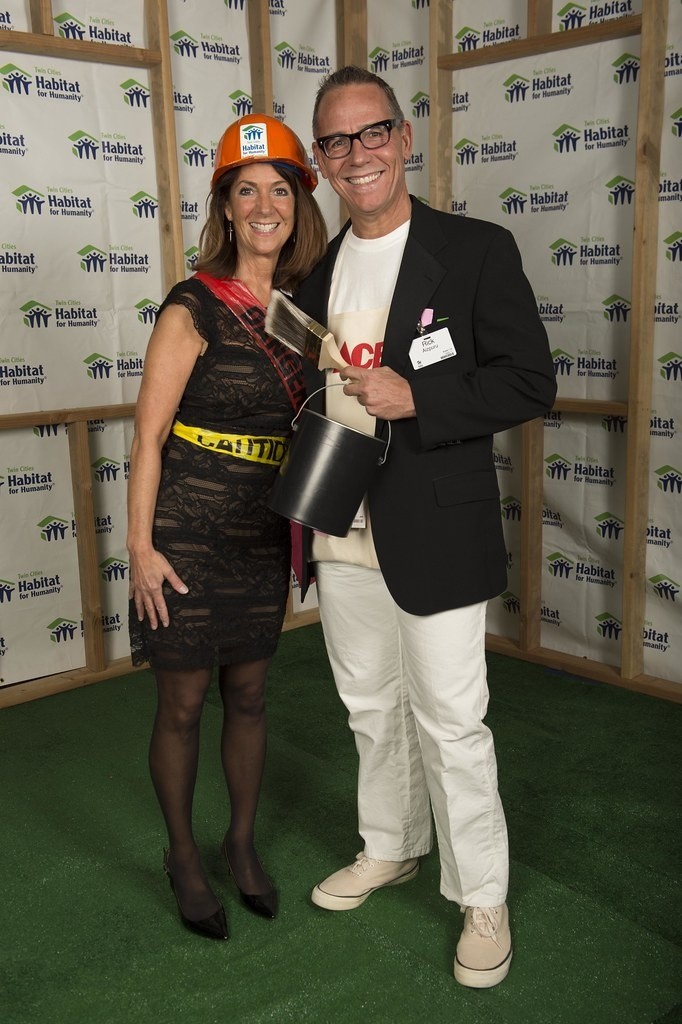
[210,113,318,194]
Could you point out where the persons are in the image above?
[126,114,328,942]
[292,66,558,988]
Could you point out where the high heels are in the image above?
[221,831,277,920]
[163,847,229,940]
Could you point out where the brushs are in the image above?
[262,288,350,372]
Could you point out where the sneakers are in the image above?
[453,902,513,989]
[311,851,420,911]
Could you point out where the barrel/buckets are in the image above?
[266,382,391,537]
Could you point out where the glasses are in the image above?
[317,119,396,160]
